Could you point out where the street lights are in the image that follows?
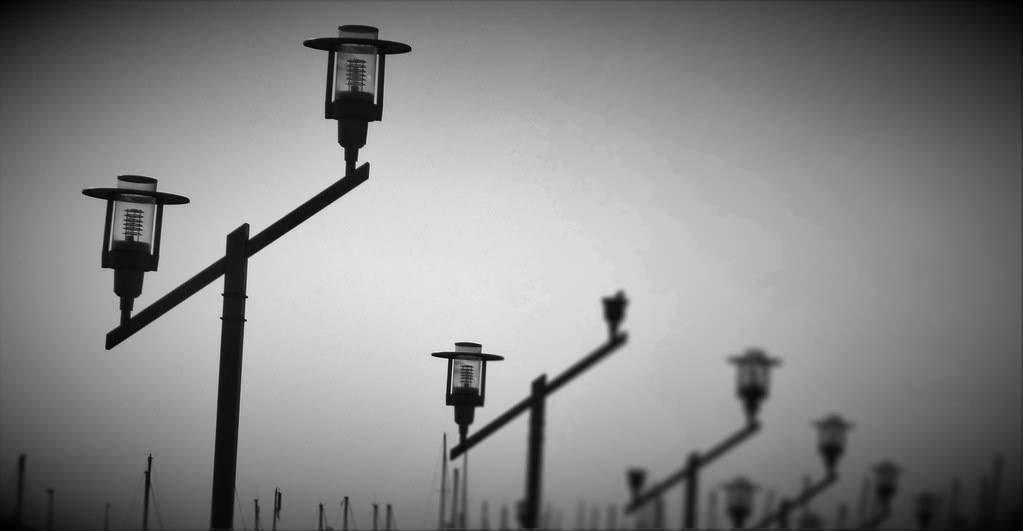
[430,293,642,530]
[78,21,415,531]
[624,347,786,531]
[720,409,856,531]
